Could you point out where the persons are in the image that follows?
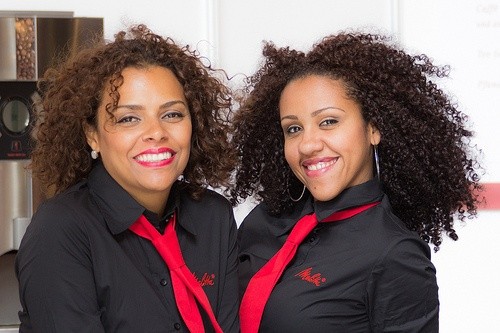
[227,31,487,333]
[15,24,241,333]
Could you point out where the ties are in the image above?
[128,212,223,333]
[239,201,379,333]
